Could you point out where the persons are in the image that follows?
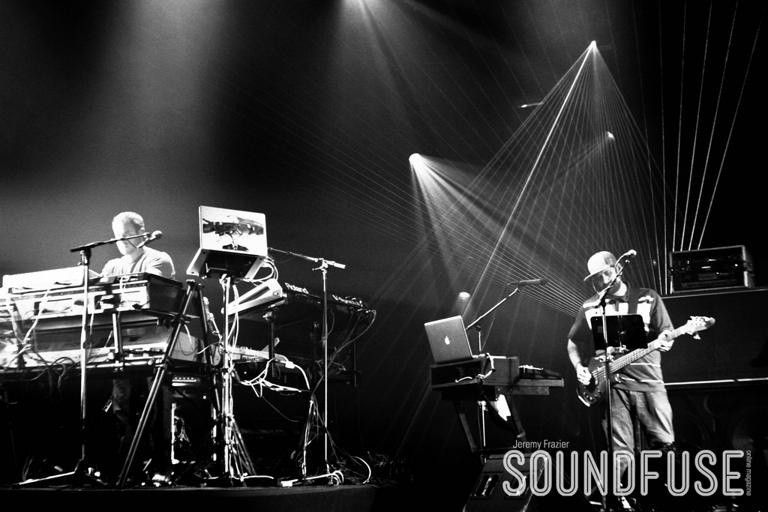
[567,251,676,481]
[99,212,177,488]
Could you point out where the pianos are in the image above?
[0,273,201,326]
[0,326,207,373]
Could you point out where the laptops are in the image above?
[423,314,507,366]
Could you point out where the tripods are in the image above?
[10,265,110,489]
[277,290,366,486]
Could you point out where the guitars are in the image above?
[576,315,716,407]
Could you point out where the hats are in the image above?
[583,251,617,283]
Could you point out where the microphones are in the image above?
[507,277,548,287]
[137,229,163,250]
[621,249,637,269]
